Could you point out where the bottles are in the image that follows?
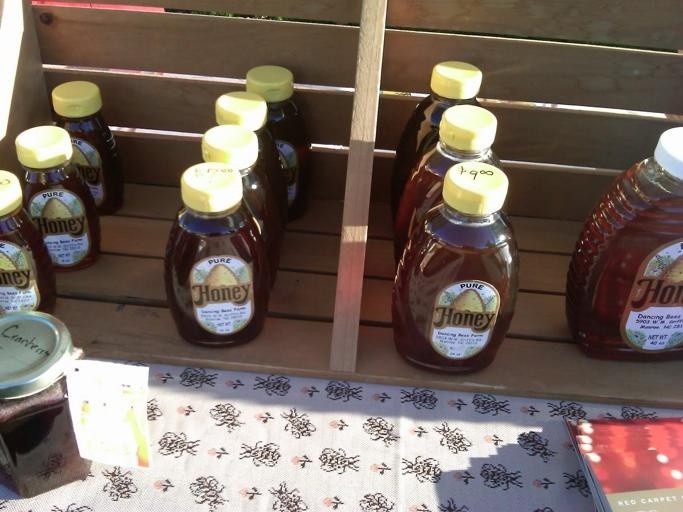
[165,161,266,349]
[388,161,526,376]
[10,125,105,270]
[201,124,276,293]
[246,63,306,220]
[392,105,501,269]
[0,168,59,319]
[561,124,683,363]
[388,56,488,213]
[213,91,289,241]
[42,79,126,216]
